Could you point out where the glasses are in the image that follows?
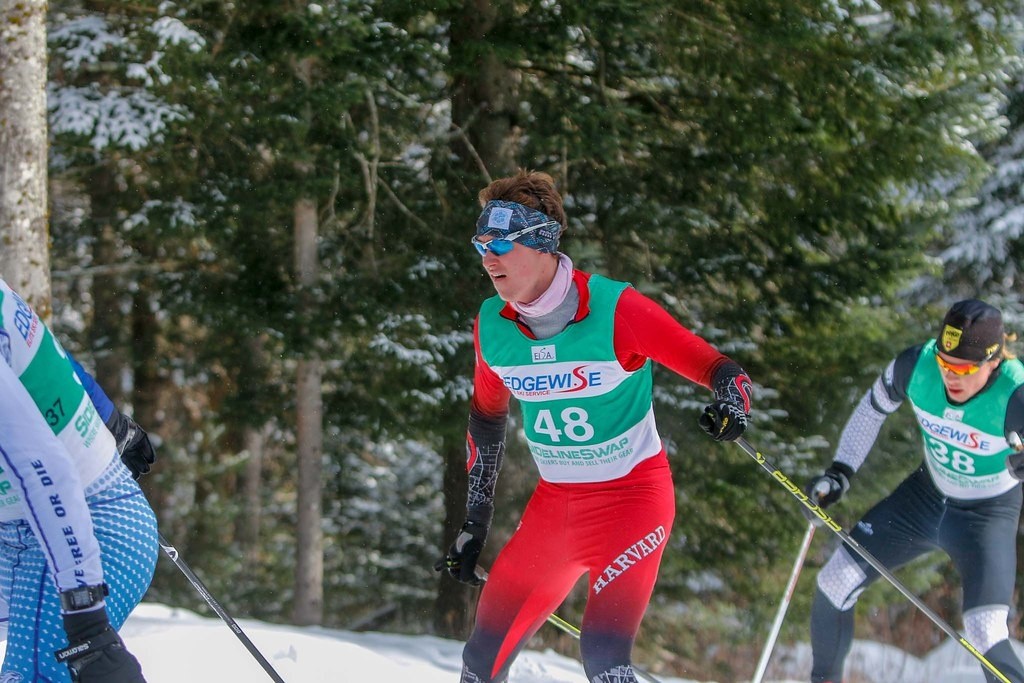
[471,221,557,256]
[933,343,1002,376]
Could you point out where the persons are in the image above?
[809,299,1023,682]
[446,170,753,683]
[0,279,159,683]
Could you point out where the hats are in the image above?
[937,299,1004,360]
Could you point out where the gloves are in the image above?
[434,519,489,588]
[107,405,155,480]
[1006,431,1024,482]
[800,469,850,527]
[54,600,147,683]
[698,400,751,442]
